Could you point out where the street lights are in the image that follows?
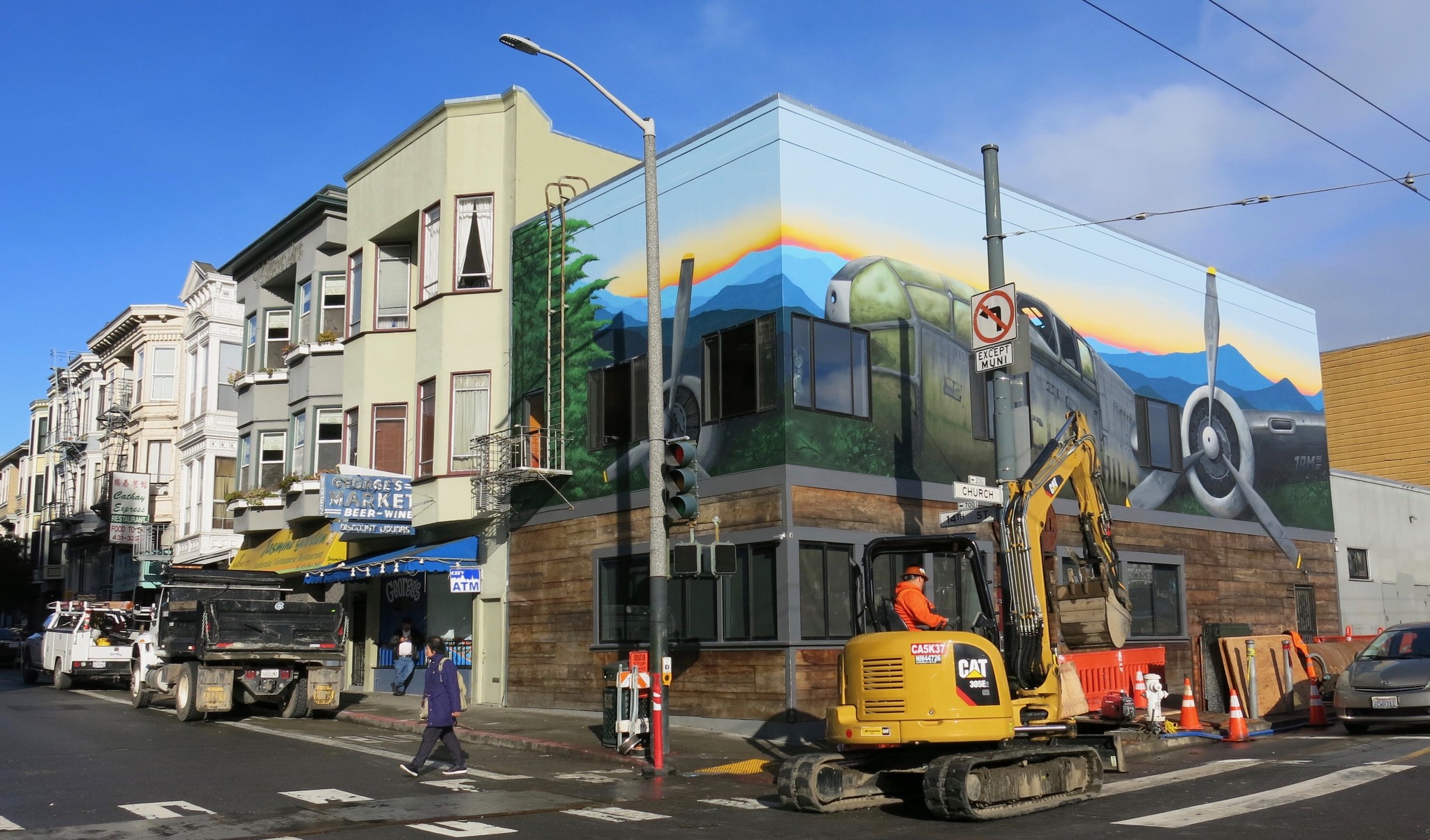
[497,31,673,757]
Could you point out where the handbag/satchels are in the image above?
[420,697,429,719]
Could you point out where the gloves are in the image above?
[946,618,959,628]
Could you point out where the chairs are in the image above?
[881,592,909,631]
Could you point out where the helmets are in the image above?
[900,566,929,582]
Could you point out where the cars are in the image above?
[1333,621,1430,736]
[0,628,32,662]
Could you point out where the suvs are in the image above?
[22,601,140,690]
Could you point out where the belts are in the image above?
[399,655,412,658]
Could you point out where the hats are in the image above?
[403,617,412,624]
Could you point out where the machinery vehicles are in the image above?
[777,409,1134,824]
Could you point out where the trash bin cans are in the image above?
[600,662,653,750]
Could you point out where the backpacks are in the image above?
[438,657,468,711]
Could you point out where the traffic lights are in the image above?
[673,544,699,577]
[662,439,698,526]
[704,542,738,575]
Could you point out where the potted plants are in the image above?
[227,367,254,390]
[281,339,310,365]
[299,468,333,491]
[309,330,345,353]
[277,470,304,495]
[253,367,289,382]
[224,484,285,511]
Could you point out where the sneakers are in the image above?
[400,764,419,777]
[396,691,404,696]
[391,682,398,694]
[442,764,467,774]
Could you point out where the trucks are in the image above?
[127,562,346,723]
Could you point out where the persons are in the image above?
[400,637,468,777]
[21,595,47,638]
[893,566,954,632]
[388,619,418,696]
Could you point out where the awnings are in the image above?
[302,535,480,585]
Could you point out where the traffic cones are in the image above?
[1134,665,1148,710]
[1219,689,1257,742]
[1303,679,1334,728]
[1176,676,1205,731]
